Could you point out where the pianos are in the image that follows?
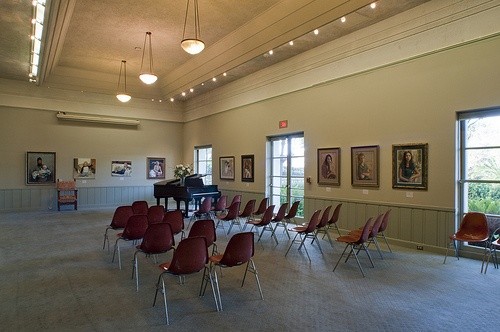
[153,174,221,218]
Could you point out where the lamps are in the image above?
[115,60,131,102]
[139,32,159,84]
[181,0,205,55]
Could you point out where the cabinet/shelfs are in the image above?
[57,179,77,210]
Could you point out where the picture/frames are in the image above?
[26,151,57,185]
[391,143,428,190]
[317,147,340,186]
[111,161,132,177]
[351,145,379,187]
[241,155,254,182]
[147,157,165,179]
[219,156,235,180]
[74,157,97,178]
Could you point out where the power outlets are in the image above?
[417,246,423,250]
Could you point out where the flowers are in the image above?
[174,164,192,177]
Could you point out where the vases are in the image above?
[180,178,185,186]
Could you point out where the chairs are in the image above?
[103,195,393,325]
[443,212,500,274]
